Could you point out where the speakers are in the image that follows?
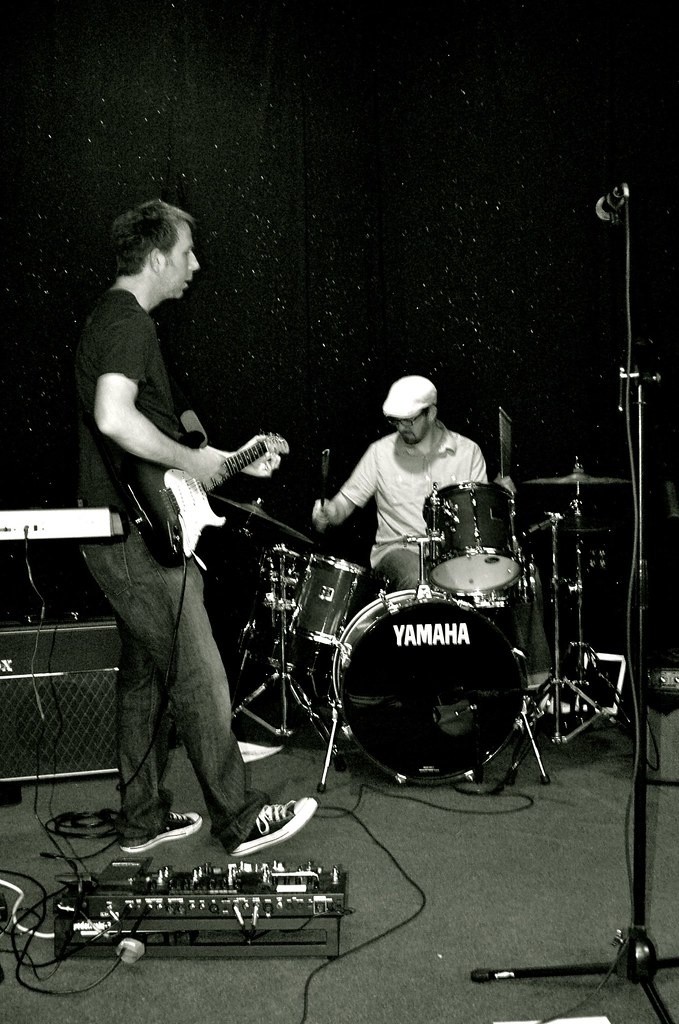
[0,615,121,780]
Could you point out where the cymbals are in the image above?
[523,460,633,486]
[206,490,315,546]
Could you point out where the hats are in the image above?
[382,375,438,418]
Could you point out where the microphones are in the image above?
[596,185,629,220]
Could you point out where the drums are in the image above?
[330,586,527,784]
[258,547,364,650]
[419,480,523,598]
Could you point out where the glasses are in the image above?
[388,409,430,426]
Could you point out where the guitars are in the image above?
[118,430,292,569]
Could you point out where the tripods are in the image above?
[230,556,348,773]
[471,199,679,1024]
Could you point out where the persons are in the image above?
[311,375,490,591]
[76,204,319,858]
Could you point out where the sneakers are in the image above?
[120,810,203,853]
[231,797,318,857]
